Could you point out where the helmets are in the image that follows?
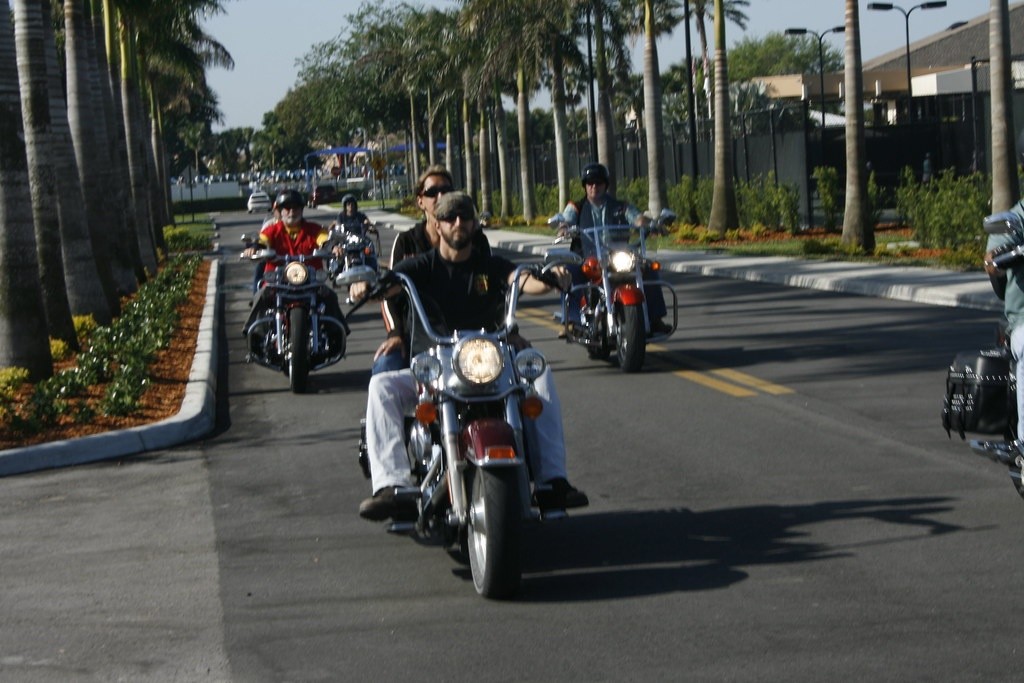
[275,190,304,207]
[342,194,356,208]
[581,164,608,179]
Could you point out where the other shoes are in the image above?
[558,325,571,337]
[651,321,673,334]
[359,486,420,523]
[536,482,589,510]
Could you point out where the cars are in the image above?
[247,191,272,214]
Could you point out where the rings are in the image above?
[382,344,386,349]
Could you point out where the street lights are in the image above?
[867,0,948,138]
[784,27,847,166]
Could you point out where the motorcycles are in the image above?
[548,207,680,374]
[941,210,1024,502]
[333,249,584,600]
[327,220,384,293]
[239,230,348,396]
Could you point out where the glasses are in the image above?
[438,209,474,222]
[421,186,454,197]
[283,204,302,209]
[586,179,607,186]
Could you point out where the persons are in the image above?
[984,200,1024,442]
[349,166,588,522]
[242,190,350,354]
[329,194,377,287]
[557,164,673,339]
